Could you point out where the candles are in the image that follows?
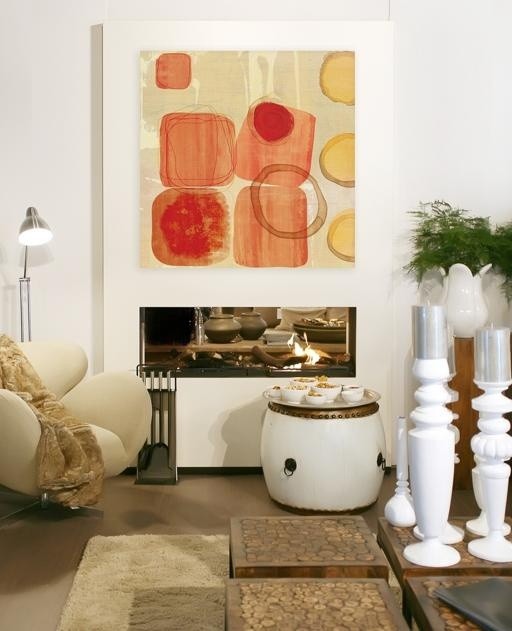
[472,322,511,383]
[412,300,450,363]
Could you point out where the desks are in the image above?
[447,338,505,492]
[293,322,346,344]
[189,338,346,369]
[259,385,387,515]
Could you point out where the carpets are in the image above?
[57,532,232,631]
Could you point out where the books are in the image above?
[431,577,512,631]
[258,328,294,346]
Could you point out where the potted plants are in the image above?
[402,197,511,338]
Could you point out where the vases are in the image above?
[202,312,267,343]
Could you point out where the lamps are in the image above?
[17,206,54,345]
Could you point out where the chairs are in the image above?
[2,340,155,525]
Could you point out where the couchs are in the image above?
[252,308,347,346]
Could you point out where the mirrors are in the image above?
[139,306,357,379]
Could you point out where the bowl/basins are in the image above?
[343,385,364,395]
[291,378,317,387]
[281,386,307,405]
[266,386,281,398]
[304,392,326,405]
[311,384,342,404]
[342,392,363,405]
